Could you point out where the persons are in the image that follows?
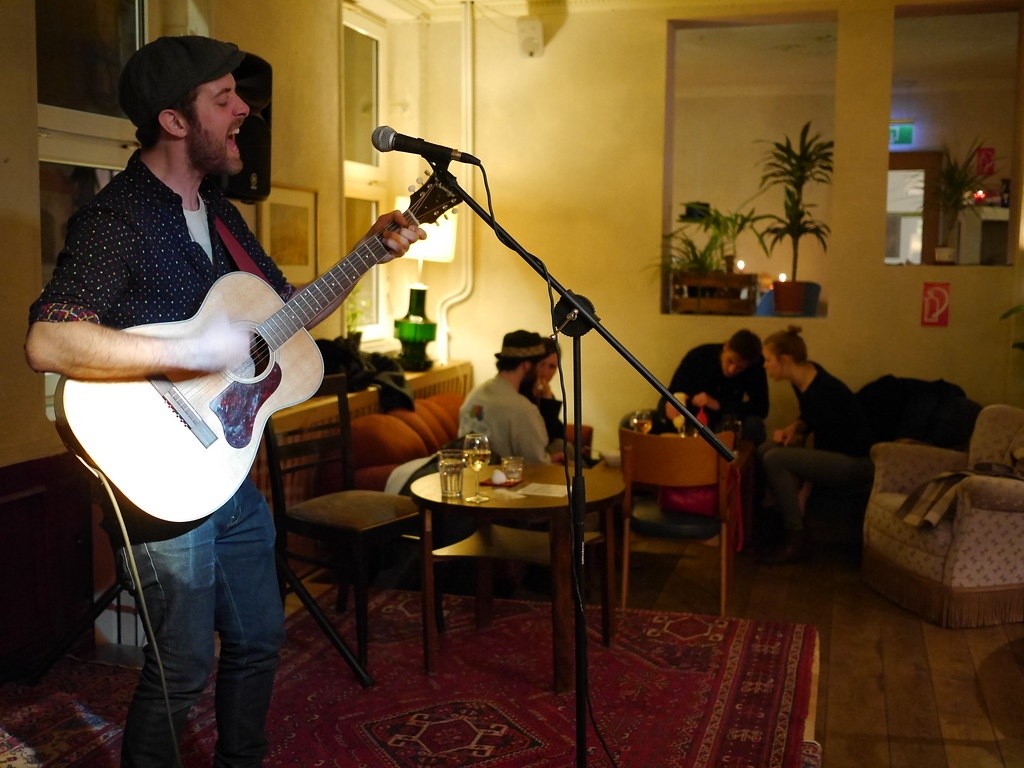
[658,328,771,510]
[24,35,427,768]
[754,324,877,565]
[456,330,590,468]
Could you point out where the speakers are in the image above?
[204,50,272,205]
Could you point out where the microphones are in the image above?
[371,126,481,166]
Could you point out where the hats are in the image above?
[118,35,243,125]
[492,330,548,361]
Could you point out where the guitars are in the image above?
[50,166,470,530]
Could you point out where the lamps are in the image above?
[383,194,460,370]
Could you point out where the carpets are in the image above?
[0,582,832,767]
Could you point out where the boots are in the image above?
[768,526,814,566]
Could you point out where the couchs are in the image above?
[349,383,592,581]
[864,404,1023,629]
[810,373,981,562]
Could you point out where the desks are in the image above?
[409,462,624,694]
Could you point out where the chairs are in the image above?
[616,427,752,618]
[261,390,426,690]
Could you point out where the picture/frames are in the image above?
[258,188,321,285]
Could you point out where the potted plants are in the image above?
[666,207,757,318]
[749,123,830,314]
[924,141,987,263]
[344,286,365,353]
